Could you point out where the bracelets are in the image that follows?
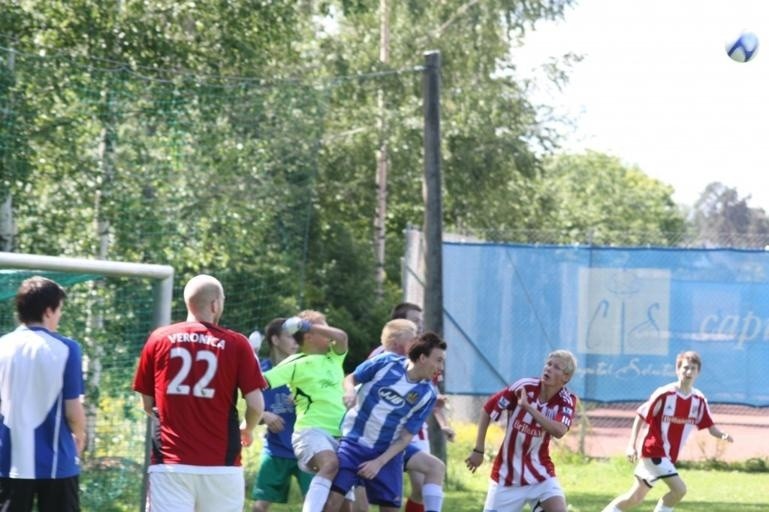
[473,448,485,454]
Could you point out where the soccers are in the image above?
[725,30,761,63]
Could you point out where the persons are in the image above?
[392,301,454,512]
[601,349,736,512]
[131,275,265,512]
[255,317,318,512]
[0,274,87,511]
[464,350,577,512]
[379,318,419,360]
[325,331,447,512]
[262,308,369,512]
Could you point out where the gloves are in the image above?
[281,315,311,336]
[246,331,265,356]
